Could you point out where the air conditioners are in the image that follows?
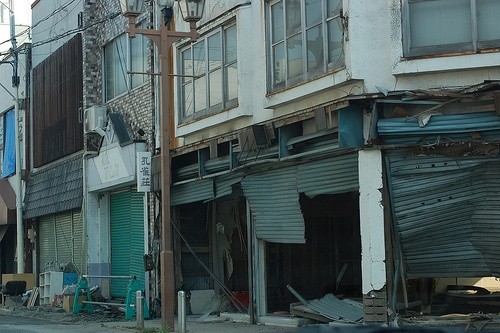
[83,104,107,134]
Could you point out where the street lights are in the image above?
[120,0,211,333]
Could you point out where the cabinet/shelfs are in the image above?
[39,270,63,307]
